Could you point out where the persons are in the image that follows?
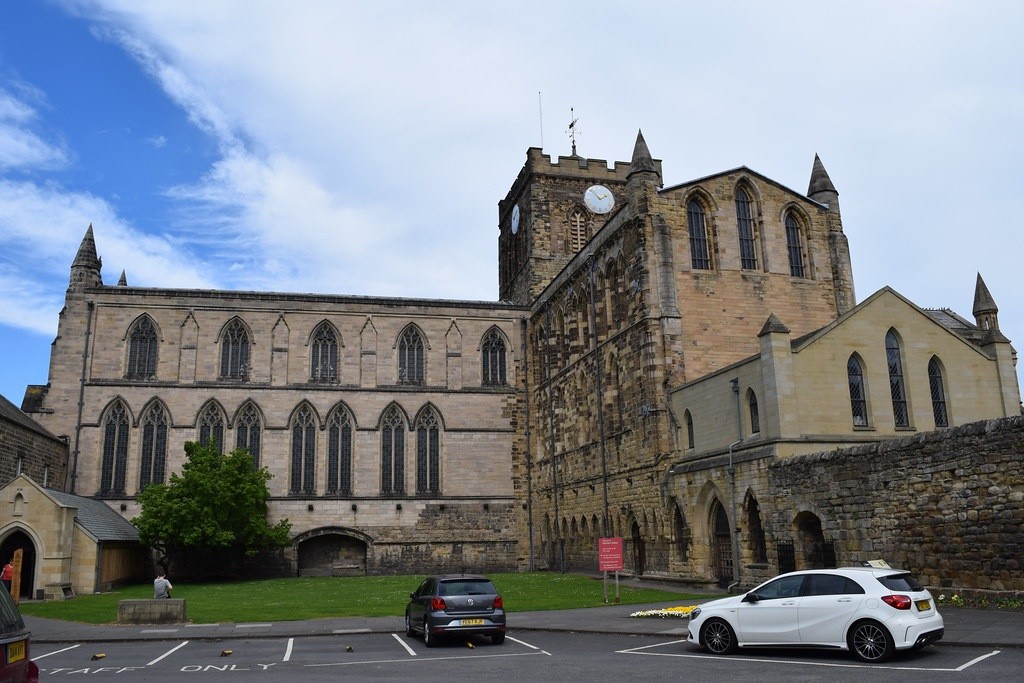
[153,568,173,599]
[0,557,13,594]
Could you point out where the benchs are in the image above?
[118,599,186,623]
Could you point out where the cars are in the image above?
[0,579,39,683]
[687,566,944,663]
[406,574,506,648]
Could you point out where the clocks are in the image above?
[584,184,614,213]
[512,205,519,234]
